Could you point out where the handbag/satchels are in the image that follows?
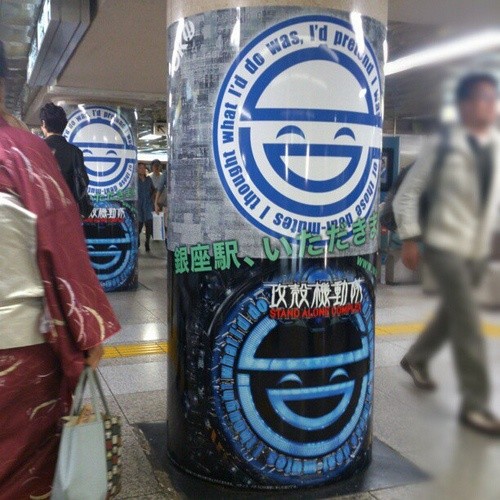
[51,364,123,500]
[381,127,450,235]
[72,144,94,221]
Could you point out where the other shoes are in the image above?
[138,236,140,248]
[144,241,151,251]
[461,409,500,437]
[401,356,436,390]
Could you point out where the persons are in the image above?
[0,38,105,500]
[137,159,168,251]
[391,73,500,437]
[40,102,95,220]
[385,158,424,284]
